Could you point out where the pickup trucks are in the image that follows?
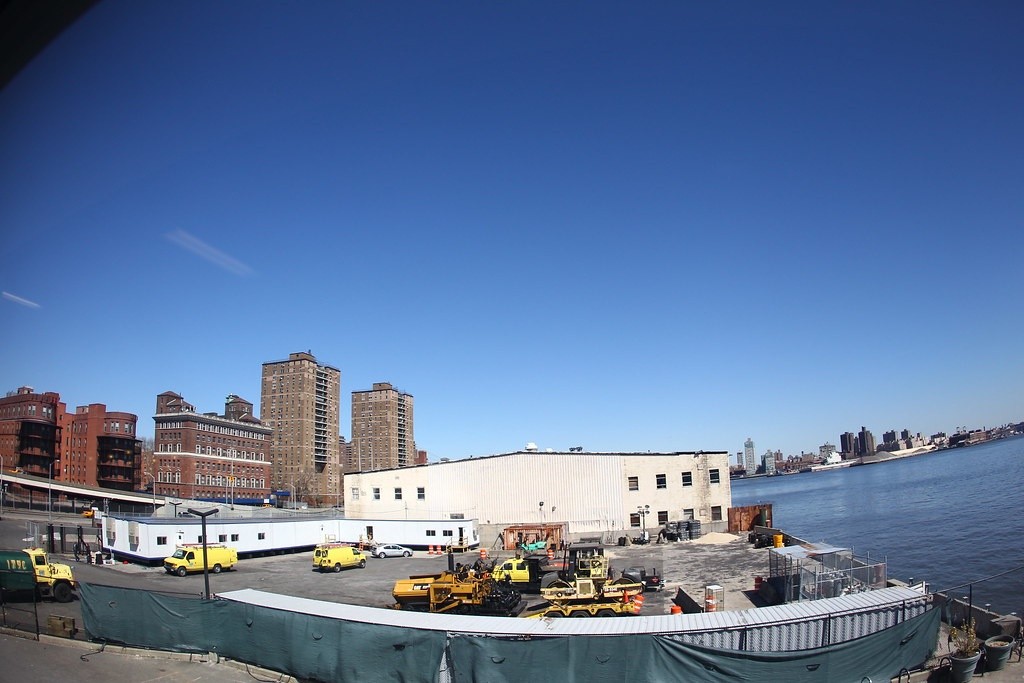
[84,507,108,518]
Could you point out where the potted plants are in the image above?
[947,619,981,683]
[984,633,1015,670]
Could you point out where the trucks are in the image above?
[163,544,237,577]
[313,542,366,572]
[0,545,75,603]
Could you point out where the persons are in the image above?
[594,584,605,603]
[455,563,461,572]
[473,557,483,571]
[503,572,513,589]
[84,543,92,565]
[459,564,464,571]
[72,541,80,562]
[656,528,670,544]
[460,563,473,573]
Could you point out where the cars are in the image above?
[372,544,413,559]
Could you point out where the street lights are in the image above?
[285,483,296,516]
[49,460,60,519]
[147,473,156,513]
[637,504,650,540]
[169,502,182,518]
[187,508,219,599]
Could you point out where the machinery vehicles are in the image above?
[386,538,665,618]
